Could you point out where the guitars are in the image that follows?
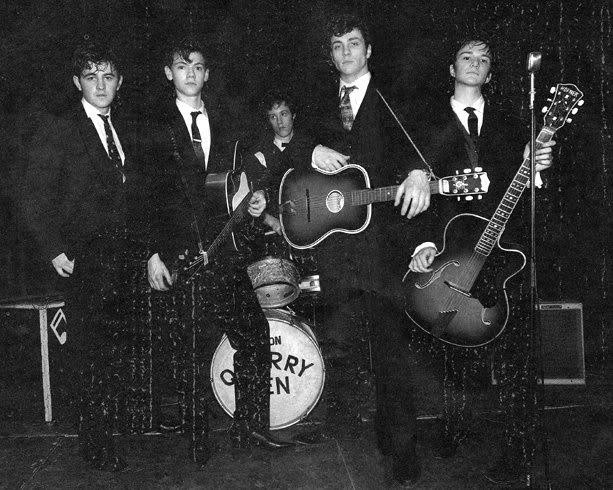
[170,138,256,297]
[279,163,491,248]
[401,84,583,348]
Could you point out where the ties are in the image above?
[189,110,205,174]
[464,105,479,150]
[338,84,355,134]
[96,113,122,178]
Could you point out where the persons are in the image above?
[20,40,143,471]
[239,96,321,178]
[290,14,438,485]
[398,31,559,488]
[131,36,297,454]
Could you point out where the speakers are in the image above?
[535,301,588,386]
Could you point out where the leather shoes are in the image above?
[430,419,461,459]
[485,446,537,486]
[119,413,187,439]
[228,421,295,454]
[391,440,424,487]
[78,433,127,476]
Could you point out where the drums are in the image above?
[210,309,327,430]
[299,274,321,300]
[246,257,301,306]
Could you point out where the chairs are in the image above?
[0,202,69,424]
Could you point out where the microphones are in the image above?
[528,51,544,73]
